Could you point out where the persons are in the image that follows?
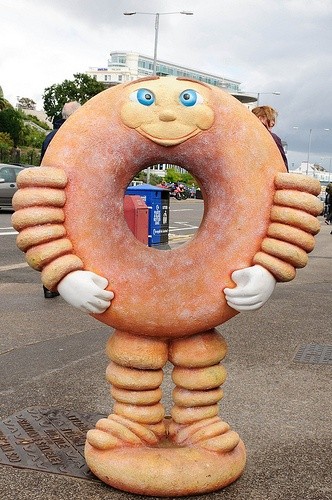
[325,192,332,225]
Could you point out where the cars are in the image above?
[0,163,29,213]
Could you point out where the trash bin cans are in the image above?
[123,195,149,246]
[159,188,170,243]
[126,182,161,244]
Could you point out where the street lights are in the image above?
[236,90,280,108]
[121,8,195,77]
[292,125,330,178]
